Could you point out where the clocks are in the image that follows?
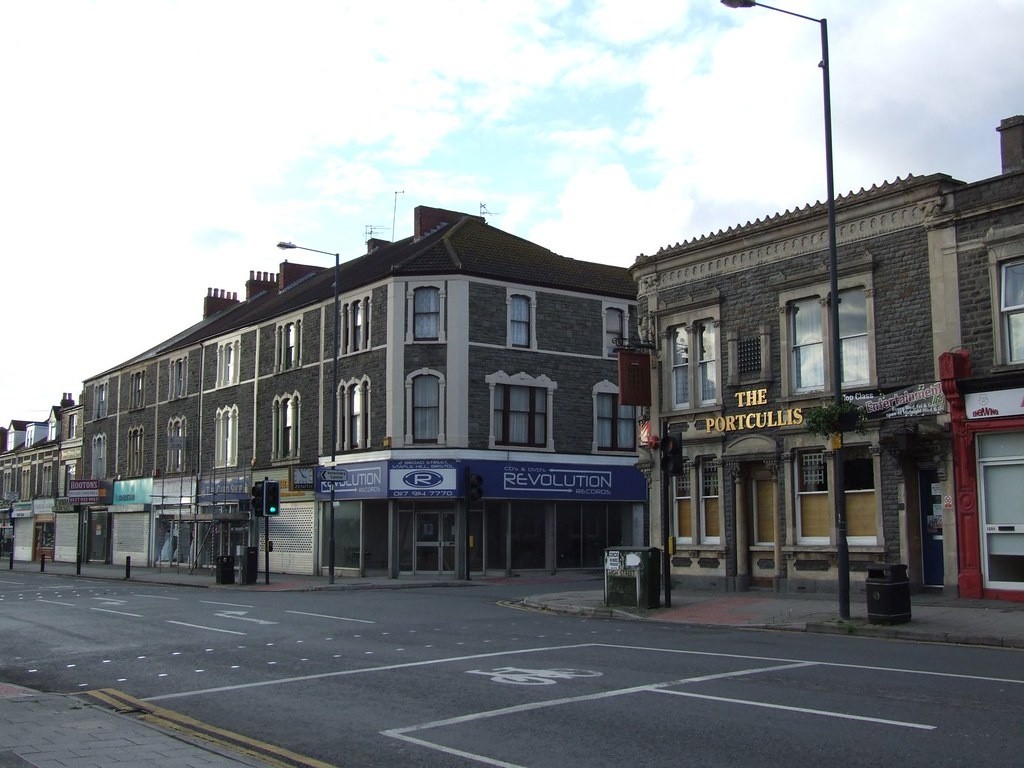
[291,466,316,492]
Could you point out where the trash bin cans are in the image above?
[602,545,660,609]
[215,555,235,585]
[864,563,913,625]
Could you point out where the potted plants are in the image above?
[803,400,871,440]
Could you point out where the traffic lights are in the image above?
[263,480,281,517]
[658,438,683,477]
[251,481,264,517]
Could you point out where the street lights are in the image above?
[722,0,851,620]
[277,241,336,585]
